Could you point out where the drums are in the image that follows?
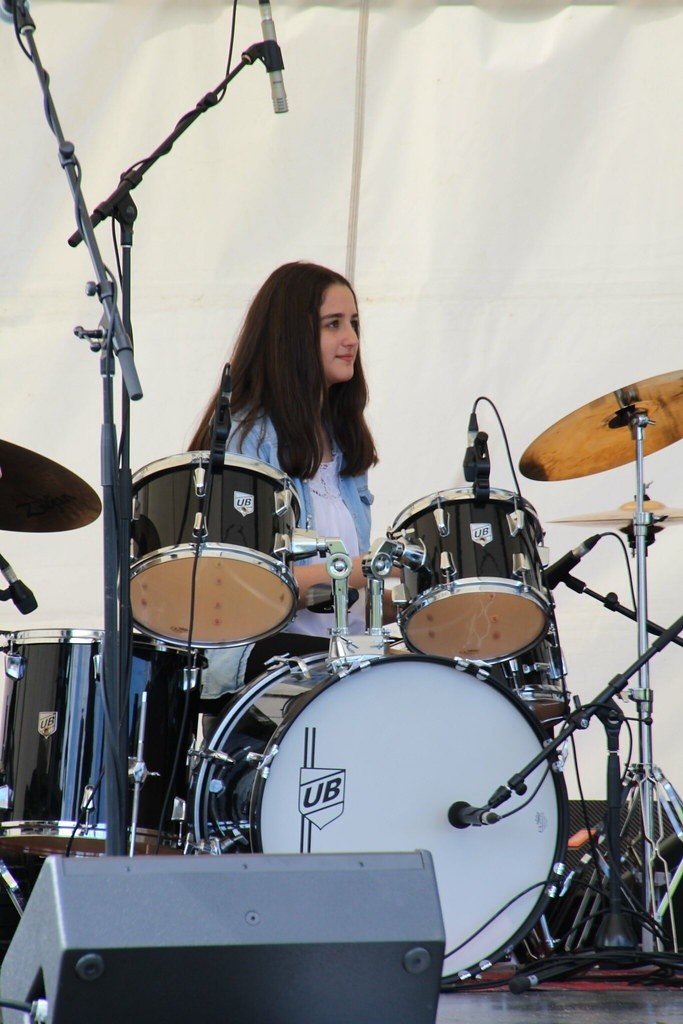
[197,637,570,999]
[386,491,554,666]
[4,627,211,862]
[129,451,303,648]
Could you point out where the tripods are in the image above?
[510,415,683,994]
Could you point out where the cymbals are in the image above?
[551,501,681,528]
[524,370,681,479]
[0,439,101,535]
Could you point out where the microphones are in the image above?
[462,412,478,482]
[259,0,288,113]
[541,533,600,590]
[447,800,500,828]
[0,553,38,615]
[0,0,30,24]
[209,365,232,439]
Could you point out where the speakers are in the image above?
[503,801,683,959]
[0,849,445,1024]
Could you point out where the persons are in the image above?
[186,261,402,743]
